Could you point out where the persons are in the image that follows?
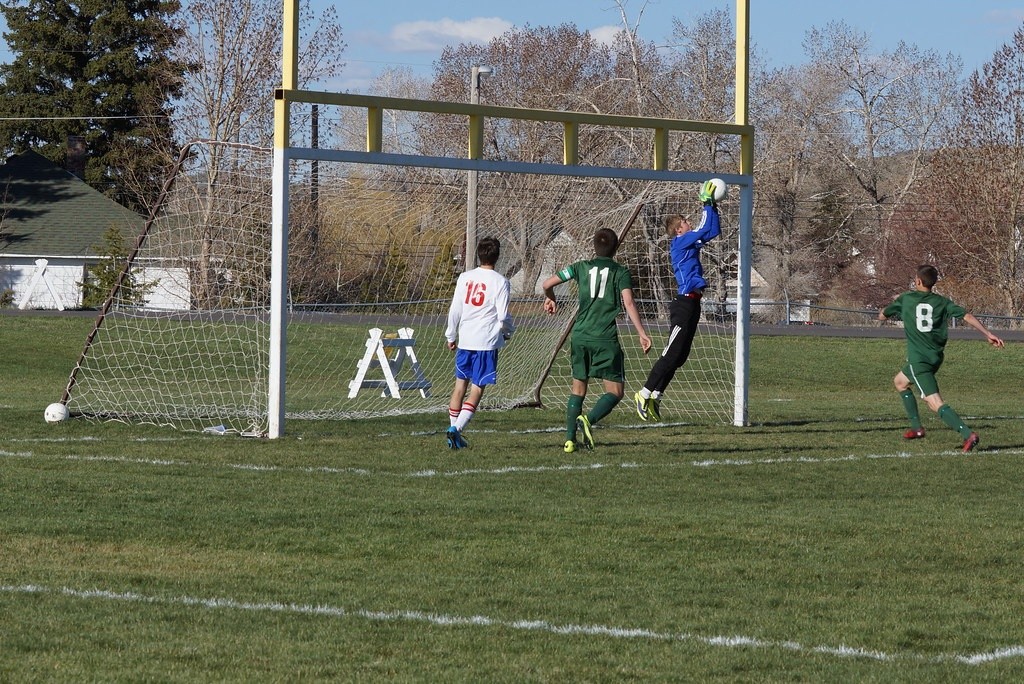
[634,181,719,423]
[542,228,652,453]
[445,237,515,451]
[878,265,1004,453]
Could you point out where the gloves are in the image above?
[696,180,716,207]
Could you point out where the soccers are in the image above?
[707,178,728,201]
[44,402,69,423]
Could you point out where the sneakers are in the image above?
[634,391,652,421]
[564,439,585,453]
[445,425,467,449]
[647,392,663,421]
[962,431,980,452]
[903,428,925,438]
[575,414,595,453]
